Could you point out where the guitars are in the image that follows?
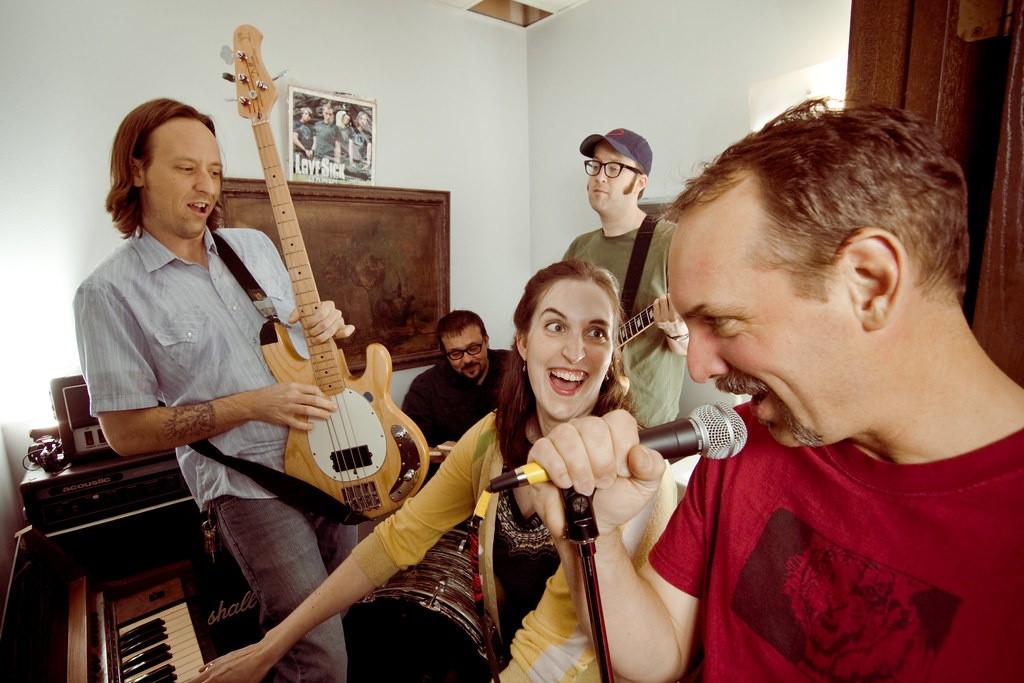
[613,297,667,347]
[217,24,428,519]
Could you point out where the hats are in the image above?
[580,128,653,176]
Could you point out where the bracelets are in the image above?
[666,333,691,342]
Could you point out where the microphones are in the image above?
[485,403,746,493]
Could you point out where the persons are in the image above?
[400,309,515,455]
[71,98,359,683]
[187,257,678,683]
[560,127,690,427]
[294,98,374,172]
[527,98,1024,680]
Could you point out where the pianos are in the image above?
[1,494,292,683]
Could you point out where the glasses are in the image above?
[584,160,643,178]
[445,338,484,360]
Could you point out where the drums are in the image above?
[344,528,501,683]
